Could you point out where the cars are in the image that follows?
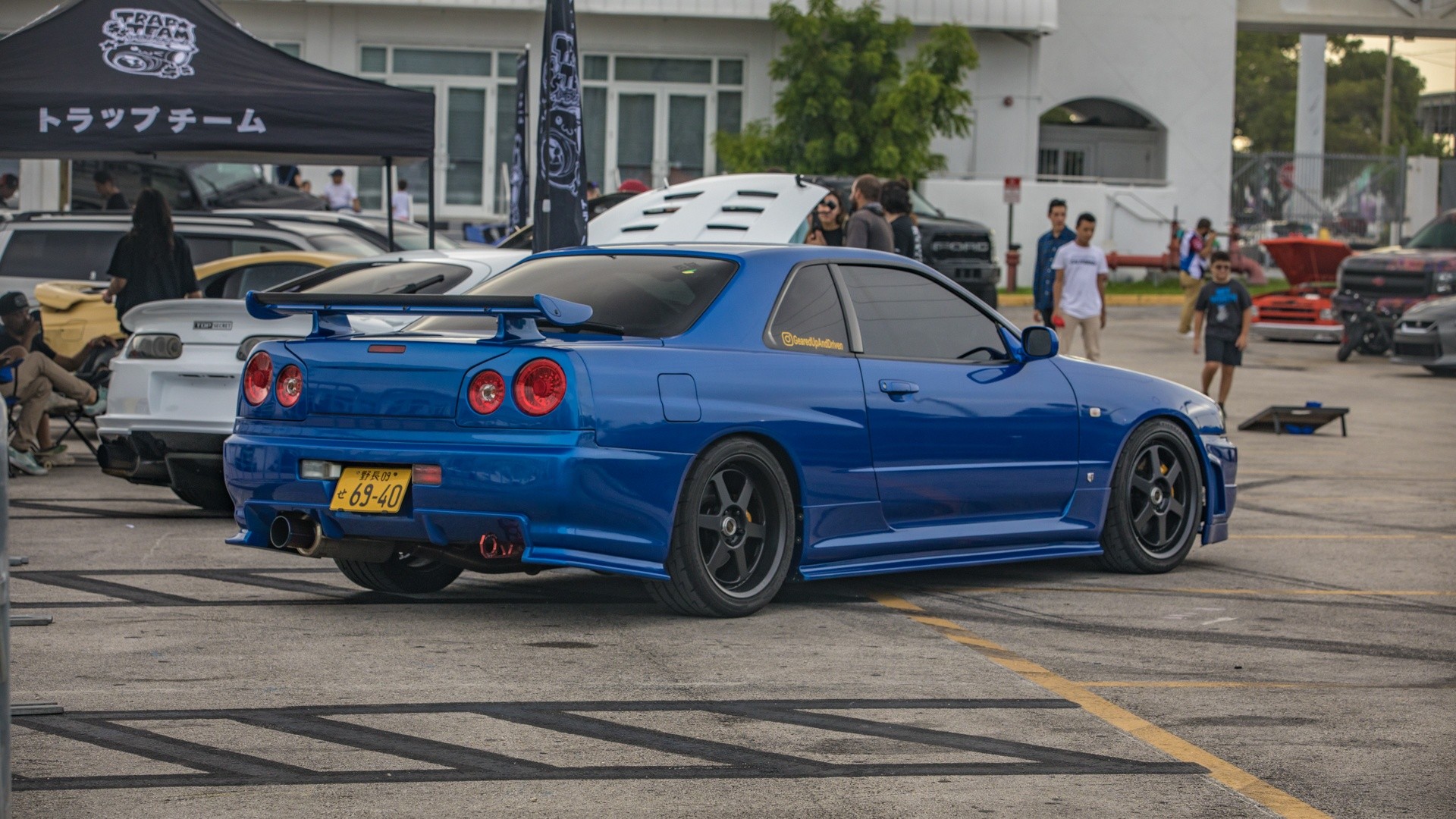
[224,240,1238,624]
[91,173,830,510]
[1241,237,1373,344]
[0,209,481,417]
[1390,294,1455,379]
[492,191,636,250]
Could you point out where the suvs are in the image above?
[1330,205,1456,358]
[810,177,1001,310]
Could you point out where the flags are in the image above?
[508,44,529,228]
[534,1,587,249]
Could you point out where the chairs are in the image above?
[0,310,97,467]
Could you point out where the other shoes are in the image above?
[8,446,48,475]
[83,386,108,416]
[42,443,68,455]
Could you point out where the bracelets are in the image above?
[87,341,94,348]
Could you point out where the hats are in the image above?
[1,290,27,316]
[329,169,343,176]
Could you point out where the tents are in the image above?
[0,0,438,257]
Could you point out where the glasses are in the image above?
[819,198,836,210]
[1216,265,1229,270]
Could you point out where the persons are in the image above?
[1051,212,1109,362]
[882,177,923,263]
[102,187,203,336]
[847,173,894,253]
[1032,199,1077,329]
[0,347,107,474]
[321,168,361,212]
[804,188,847,247]
[93,170,128,210]
[881,188,916,259]
[301,180,311,193]
[0,171,18,206]
[1196,252,1253,417]
[277,164,301,189]
[0,291,119,467]
[392,179,412,221]
[1177,218,1217,340]
